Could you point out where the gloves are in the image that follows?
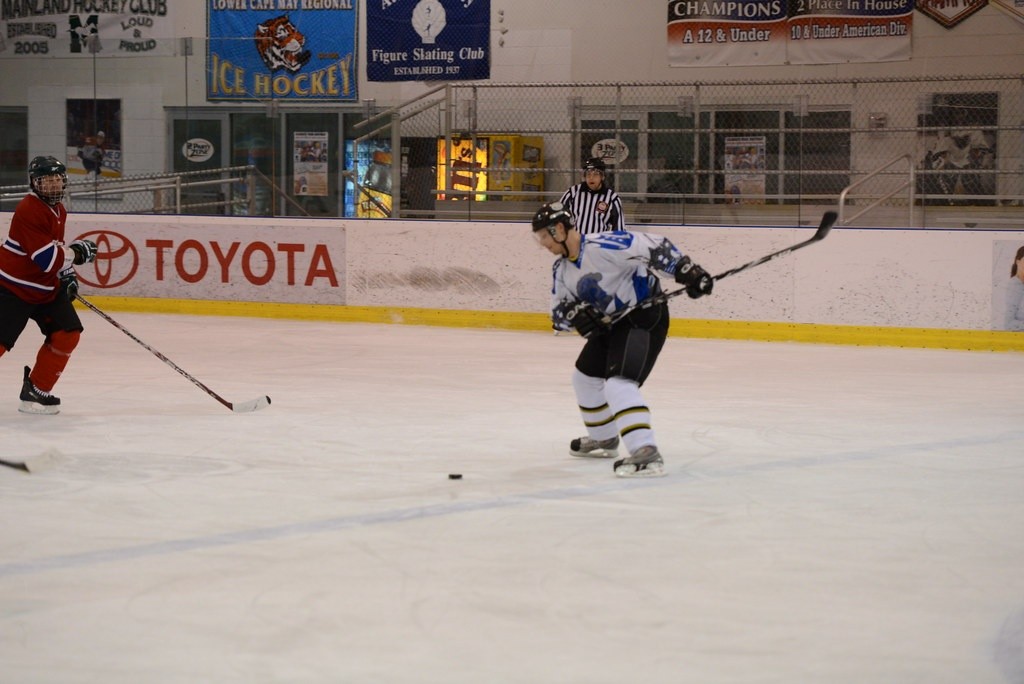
[69,240,98,266]
[674,255,714,299]
[54,275,78,303]
[563,301,606,337]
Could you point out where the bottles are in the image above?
[355,151,392,218]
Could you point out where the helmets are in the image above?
[580,157,609,171]
[532,202,578,258]
[27,155,74,206]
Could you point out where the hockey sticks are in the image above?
[607,211,840,322]
[73,291,271,414]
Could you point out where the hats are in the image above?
[97,131,106,137]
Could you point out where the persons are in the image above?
[1002,245,1023,332]
[299,141,328,162]
[926,123,991,206]
[550,158,625,337]
[532,201,713,476]
[77,131,106,188]
[0,155,98,411]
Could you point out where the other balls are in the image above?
[449,473,463,480]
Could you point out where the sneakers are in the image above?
[19,366,62,413]
[613,445,668,479]
[570,436,620,458]
